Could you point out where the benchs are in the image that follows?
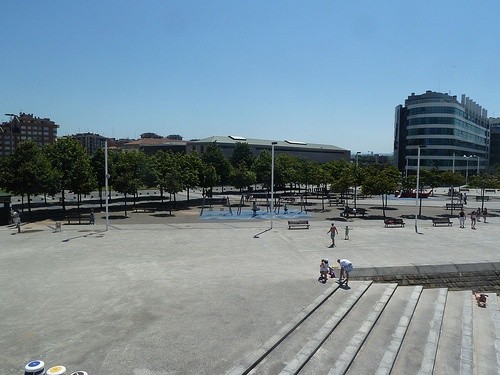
[328,200,345,206]
[384,218,405,228]
[432,217,453,227]
[65,208,95,225]
[287,220,310,229]
[476,196,490,202]
[446,203,463,210]
[343,208,366,217]
[133,203,158,213]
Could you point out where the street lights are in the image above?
[354,151,362,218]
[270,141,278,229]
[100,137,109,231]
[415,145,427,232]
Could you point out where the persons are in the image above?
[471,211,477,229]
[463,193,468,204]
[477,208,481,222]
[327,223,338,246]
[318,259,334,283]
[457,209,466,227]
[343,225,353,240]
[251,202,258,216]
[11,210,21,227]
[89,208,94,223]
[283,203,288,213]
[483,208,488,222]
[343,205,353,217]
[384,218,396,227]
[474,294,489,307]
[337,259,353,287]
[221,196,227,205]
